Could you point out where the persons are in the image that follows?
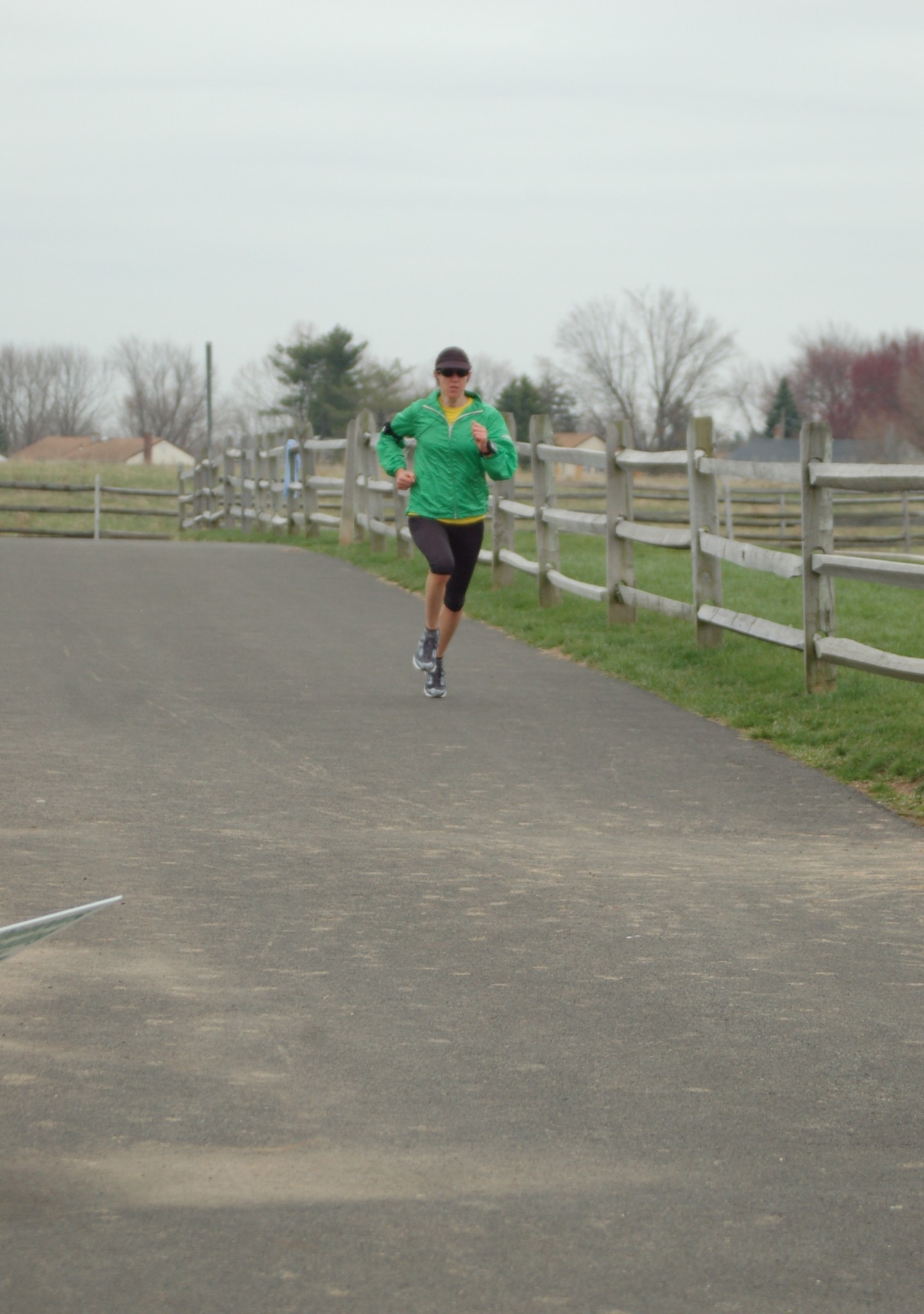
[376,347,518,698]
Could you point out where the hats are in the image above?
[435,346,472,370]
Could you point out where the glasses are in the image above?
[437,368,469,377]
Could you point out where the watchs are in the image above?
[479,440,498,458]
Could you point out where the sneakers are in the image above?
[423,660,447,698]
[412,627,440,673]
